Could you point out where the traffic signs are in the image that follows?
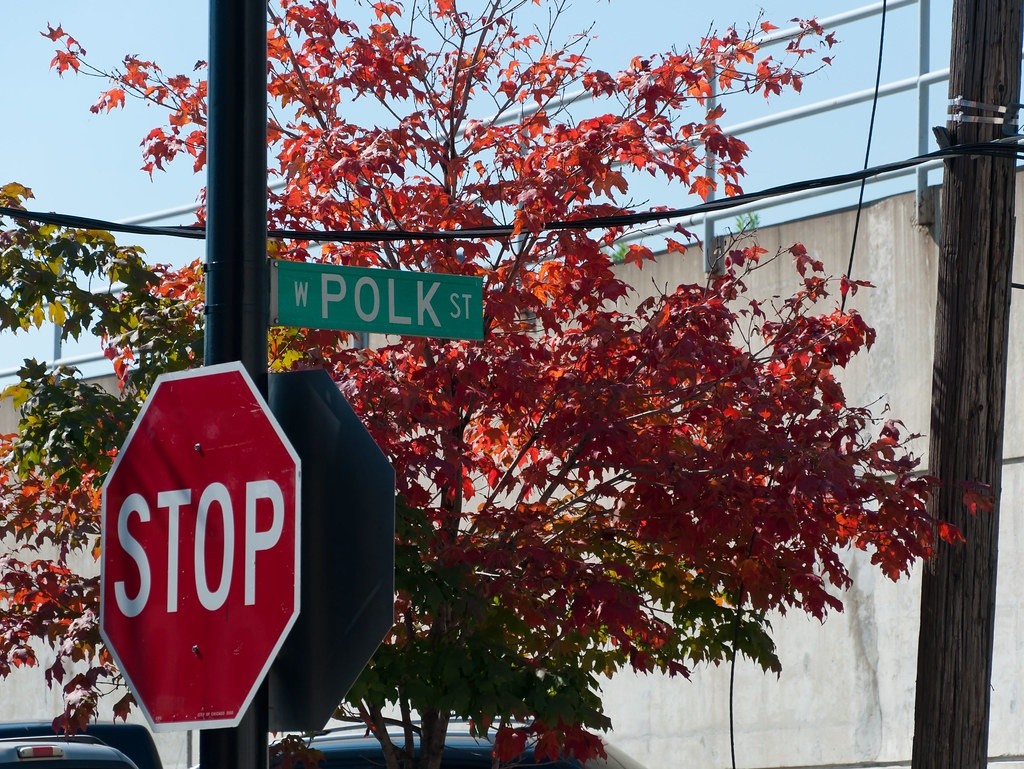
[270,261,490,346]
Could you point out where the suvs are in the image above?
[0,715,645,769]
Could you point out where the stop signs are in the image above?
[97,365,300,730]
